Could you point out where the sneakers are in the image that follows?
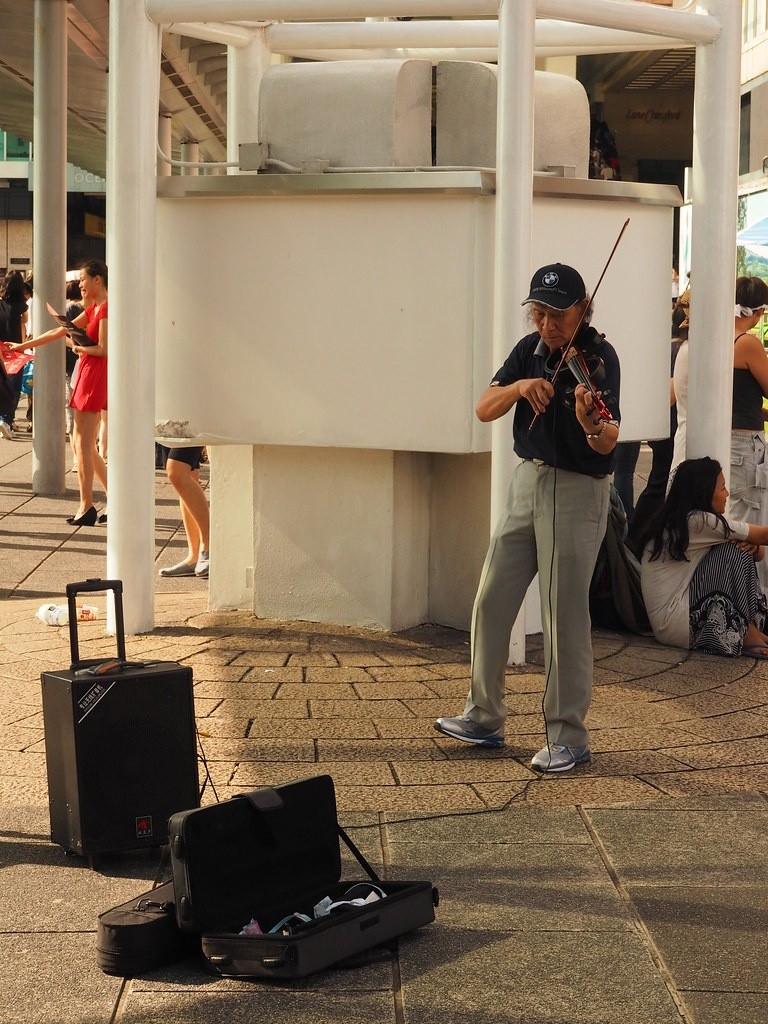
[532,741,592,773]
[433,715,505,747]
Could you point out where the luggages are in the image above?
[40,577,200,856]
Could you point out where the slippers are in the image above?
[742,644,768,659]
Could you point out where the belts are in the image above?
[525,458,605,478]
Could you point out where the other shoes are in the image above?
[159,561,195,576]
[72,463,78,472]
[0,421,12,440]
[194,550,210,577]
[27,426,32,433]
[12,423,19,430]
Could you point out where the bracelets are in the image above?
[585,422,607,440]
[72,346,79,354]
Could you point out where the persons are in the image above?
[0,259,210,578]
[631,266,768,593]
[433,262,622,773]
[634,455,768,658]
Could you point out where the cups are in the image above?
[76,605,99,622]
[38,603,69,626]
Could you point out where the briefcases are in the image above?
[169,775,436,981]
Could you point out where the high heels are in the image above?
[98,514,107,523]
[65,506,97,527]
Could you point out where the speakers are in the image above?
[41,659,200,855]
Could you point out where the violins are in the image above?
[543,324,614,423]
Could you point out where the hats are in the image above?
[521,263,586,311]
